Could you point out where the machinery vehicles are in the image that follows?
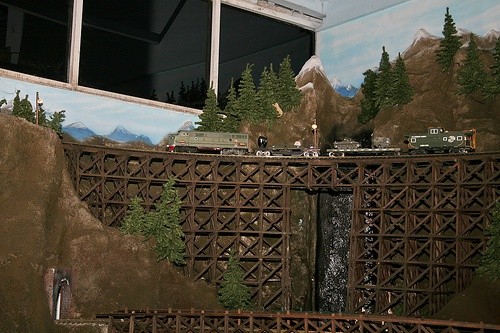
[403,126,478,155]
[255,136,321,158]
[168,131,249,156]
[324,134,402,155]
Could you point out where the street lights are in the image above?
[35,91,44,125]
[312,124,317,150]
